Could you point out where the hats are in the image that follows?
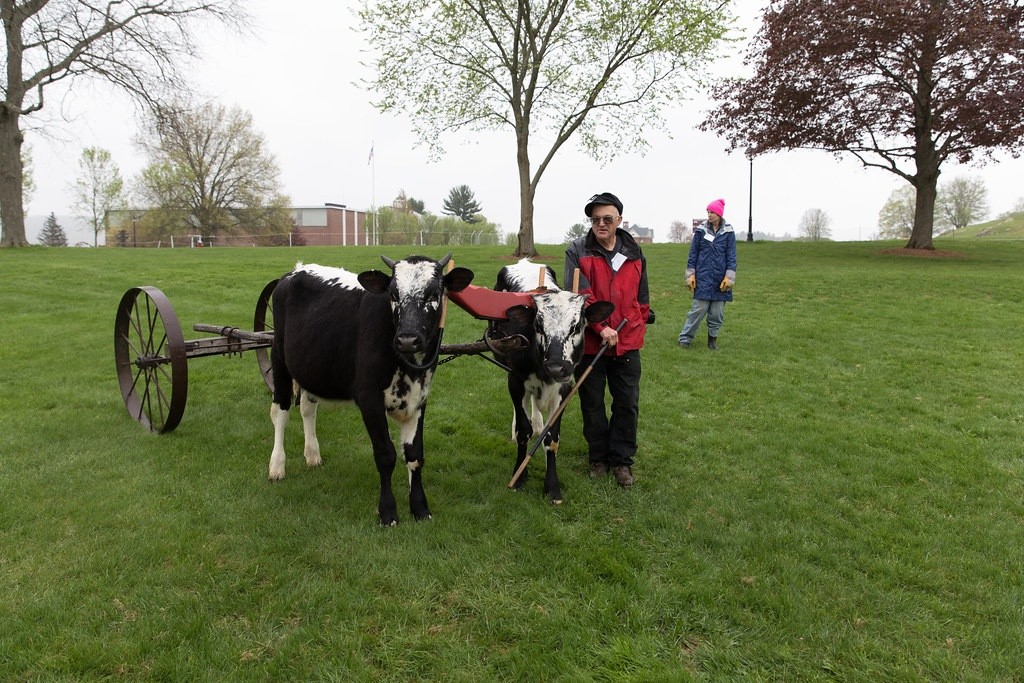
[706,199,725,218]
[585,192,623,217]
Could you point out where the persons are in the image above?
[564,192,655,486]
[679,198,738,350]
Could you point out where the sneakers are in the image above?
[590,460,607,479]
[613,465,634,487]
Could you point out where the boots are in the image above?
[680,338,690,349]
[708,332,718,350]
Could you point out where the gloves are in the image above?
[720,277,734,292]
[687,274,696,290]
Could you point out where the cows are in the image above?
[267,250,475,527]
[487,255,615,504]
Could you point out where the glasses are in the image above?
[590,215,619,225]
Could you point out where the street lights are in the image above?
[744,144,756,241]
[133,218,138,247]
